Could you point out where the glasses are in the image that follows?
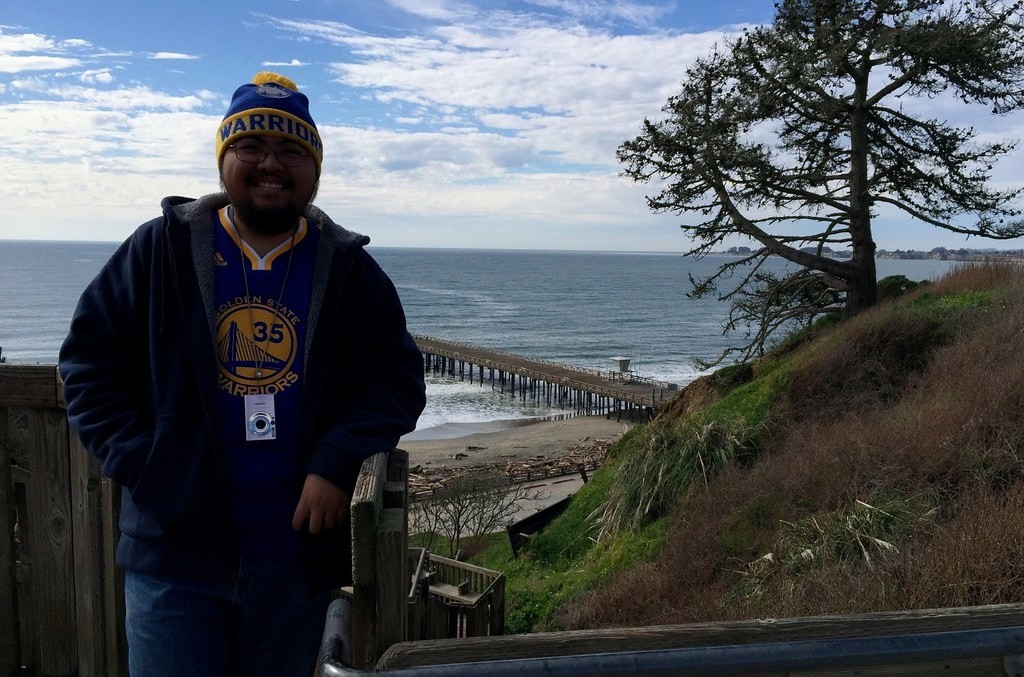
[228,140,312,167]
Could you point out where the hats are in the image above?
[216,72,323,181]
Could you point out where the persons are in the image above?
[58,71,426,677]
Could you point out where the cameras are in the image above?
[245,394,276,440]
